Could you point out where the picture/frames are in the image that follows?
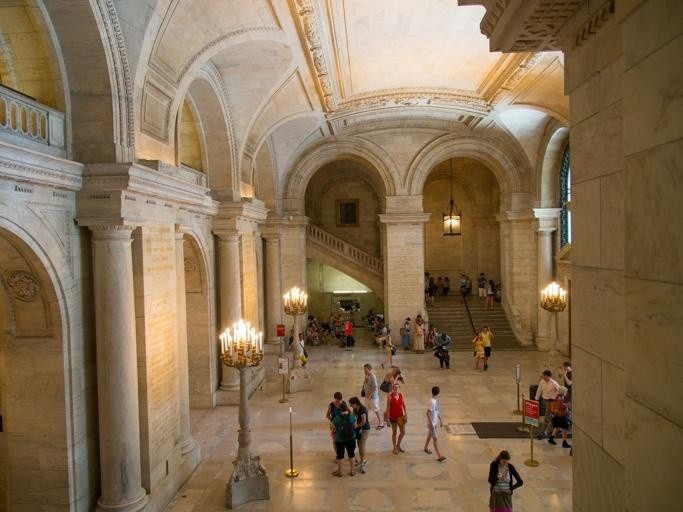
[334,198,360,227]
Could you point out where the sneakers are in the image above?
[547,438,557,445]
[381,363,384,369]
[562,440,571,448]
[424,447,433,455]
[475,365,489,372]
[536,432,544,440]
[439,365,450,368]
[332,470,343,477]
[351,456,368,476]
[392,447,405,455]
[436,456,447,461]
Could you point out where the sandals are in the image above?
[375,424,385,430]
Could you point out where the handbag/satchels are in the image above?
[361,381,367,398]
[433,348,449,361]
[388,336,397,355]
[299,343,309,358]
[379,380,391,393]
[397,407,408,426]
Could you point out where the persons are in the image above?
[460,274,473,297]
[424,386,447,461]
[367,309,396,370]
[401,315,451,370]
[327,363,408,477]
[536,362,573,448]
[488,450,523,512]
[307,312,353,340]
[296,333,307,367]
[478,272,496,311]
[425,272,450,307]
[472,326,494,372]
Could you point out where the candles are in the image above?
[219,318,263,356]
[284,287,308,307]
[541,282,567,303]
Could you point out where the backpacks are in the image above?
[337,412,354,441]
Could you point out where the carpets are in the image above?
[471,422,572,439]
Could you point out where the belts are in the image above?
[543,399,555,402]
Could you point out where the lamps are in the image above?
[442,159,462,237]
